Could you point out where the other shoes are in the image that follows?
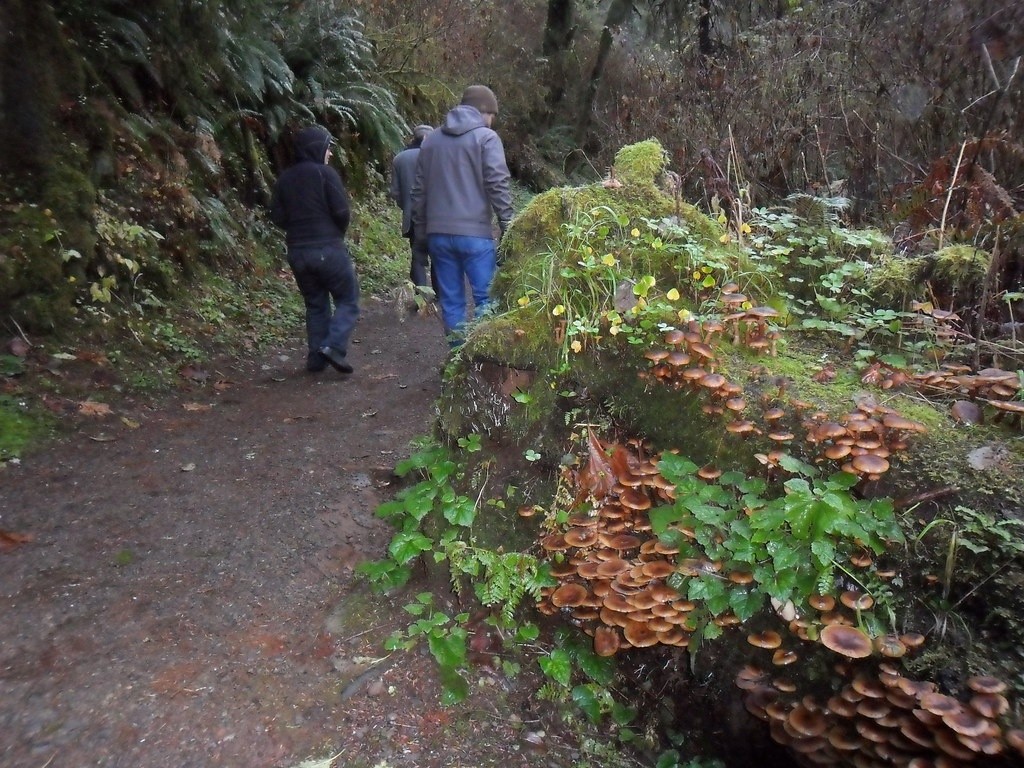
[308,352,328,371]
[318,345,355,373]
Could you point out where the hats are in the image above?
[414,125,433,140]
[462,85,498,116]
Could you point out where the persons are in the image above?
[388,124,438,312]
[272,127,360,374]
[409,85,517,349]
